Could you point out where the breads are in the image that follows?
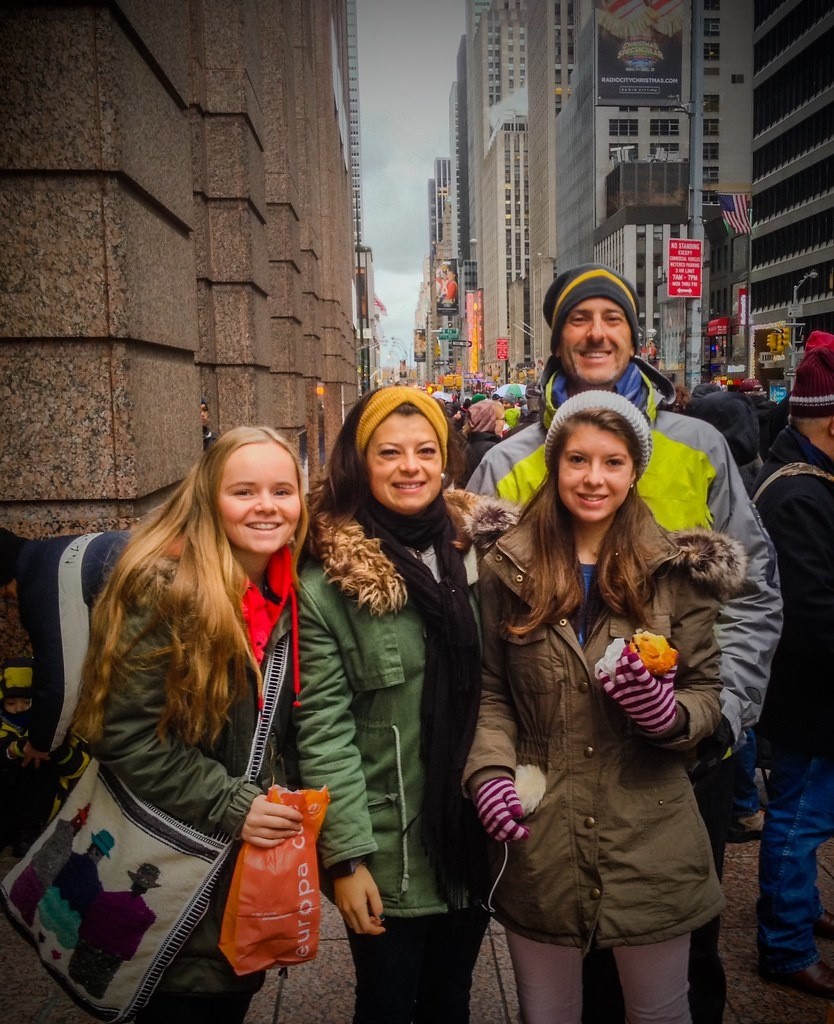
[628,631,678,675]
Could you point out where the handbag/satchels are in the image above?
[218,785,330,976]
[0,758,232,1024]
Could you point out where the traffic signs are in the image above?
[452,340,472,347]
[669,238,702,297]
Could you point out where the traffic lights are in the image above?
[766,328,789,353]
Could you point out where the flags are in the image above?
[716,193,753,235]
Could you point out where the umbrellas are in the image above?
[491,383,526,397]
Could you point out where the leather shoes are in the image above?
[814,910,834,937]
[755,958,834,994]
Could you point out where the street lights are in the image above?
[790,273,818,392]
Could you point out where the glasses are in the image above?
[201,406,209,412]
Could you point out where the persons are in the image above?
[0,263,834,1024]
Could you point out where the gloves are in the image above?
[475,778,530,843]
[598,645,677,734]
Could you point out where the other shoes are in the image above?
[730,809,765,834]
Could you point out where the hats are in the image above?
[542,264,641,357]
[0,527,18,585]
[544,389,655,479]
[788,330,834,417]
